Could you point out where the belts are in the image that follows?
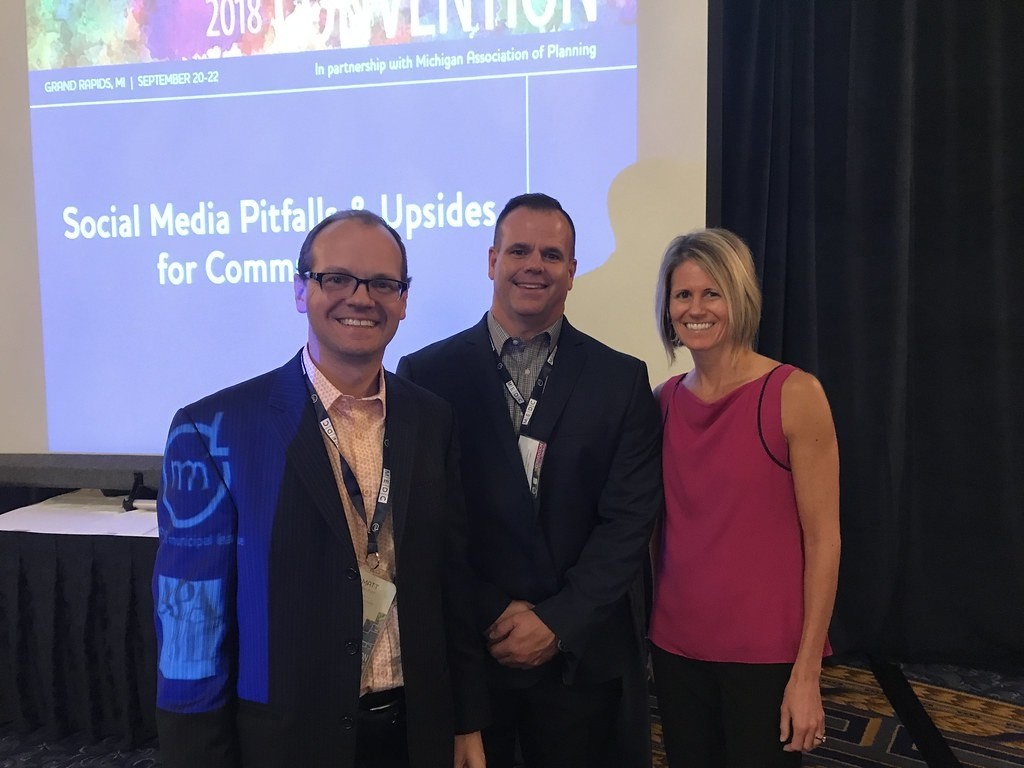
[357,698,407,736]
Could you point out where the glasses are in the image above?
[295,270,412,304]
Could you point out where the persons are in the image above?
[647,230,843,768]
[395,193,663,768]
[152,209,482,768]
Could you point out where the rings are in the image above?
[815,736,826,742]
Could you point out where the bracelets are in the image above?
[557,637,571,654]
